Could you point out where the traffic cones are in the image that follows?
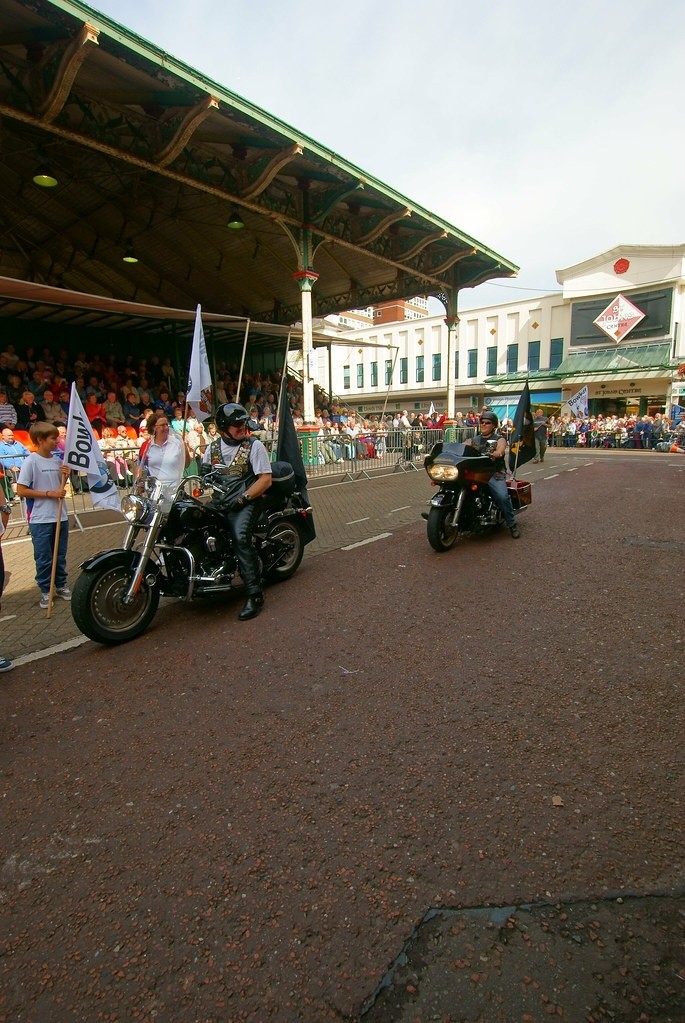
[669,443,685,453]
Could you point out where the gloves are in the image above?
[228,494,249,511]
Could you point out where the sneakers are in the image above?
[55,587,72,601]
[0,653,14,673]
[39,591,53,608]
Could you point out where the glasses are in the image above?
[481,421,494,424]
[226,419,246,428]
[154,423,168,428]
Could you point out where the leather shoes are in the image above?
[238,591,265,621]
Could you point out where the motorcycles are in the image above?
[71,460,317,646]
[421,403,532,552]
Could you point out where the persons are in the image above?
[461,411,520,538]
[270,365,390,465]
[481,405,488,414]
[132,413,191,566]
[669,411,685,446]
[197,403,273,619]
[0,484,14,674]
[16,420,72,607]
[403,431,425,462]
[548,413,674,450]
[0,342,139,505]
[137,356,273,476]
[363,410,479,451]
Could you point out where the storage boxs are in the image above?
[261,461,296,493]
[506,480,532,509]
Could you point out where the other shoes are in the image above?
[126,470,133,475]
[118,473,124,479]
[376,456,384,459]
[351,458,355,461]
[364,455,370,460]
[328,461,332,464]
[6,501,13,507]
[533,460,539,464]
[13,496,20,503]
[359,456,363,459]
[540,457,544,462]
[338,458,344,463]
[335,460,338,464]
[510,524,520,538]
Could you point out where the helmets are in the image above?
[214,402,249,430]
[480,411,498,428]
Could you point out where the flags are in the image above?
[509,380,537,472]
[63,383,122,513]
[186,305,213,421]
[566,385,589,419]
[277,375,310,507]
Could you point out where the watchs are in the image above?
[242,491,251,501]
[0,505,11,514]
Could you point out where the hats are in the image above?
[178,392,184,396]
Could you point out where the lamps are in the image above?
[122,238,139,263]
[33,155,58,187]
[601,385,605,388]
[226,205,246,229]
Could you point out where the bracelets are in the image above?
[45,491,50,498]
